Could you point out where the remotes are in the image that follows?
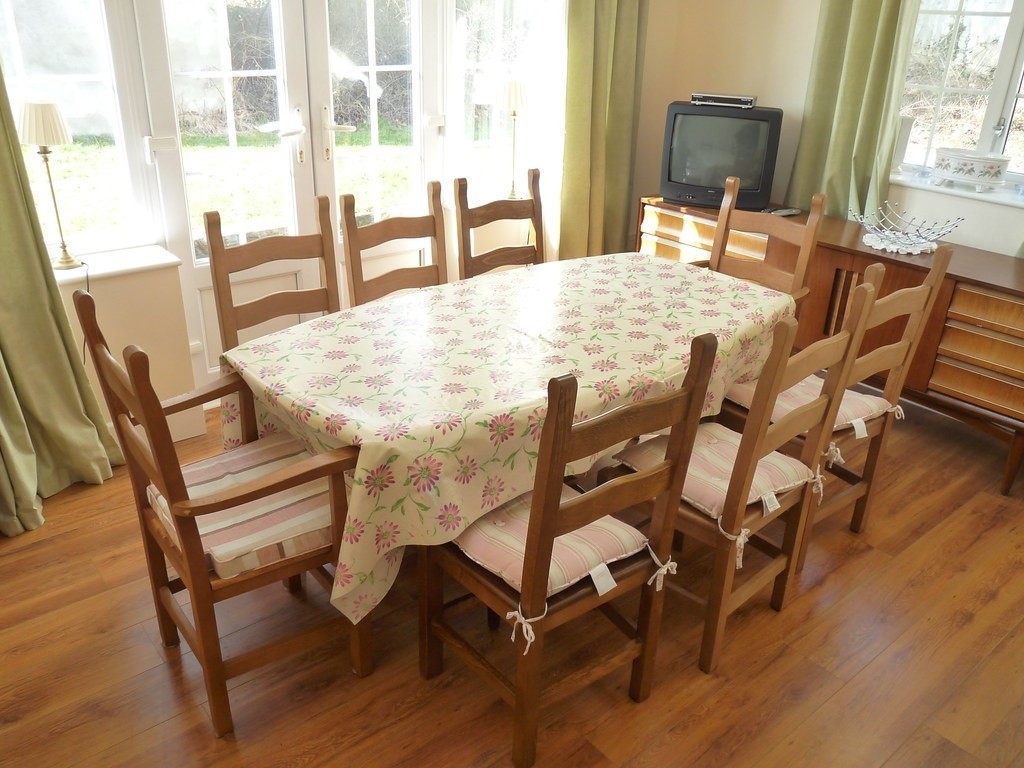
[761,209,803,217]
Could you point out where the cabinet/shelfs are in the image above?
[635,194,1024,497]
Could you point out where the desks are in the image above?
[219,252,797,634]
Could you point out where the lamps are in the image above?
[15,102,83,271]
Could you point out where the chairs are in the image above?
[595,282,876,675]
[420,333,717,768]
[686,176,828,320]
[703,244,954,573]
[72,289,375,738]
[203,196,340,352]
[340,179,448,307]
[453,168,547,279]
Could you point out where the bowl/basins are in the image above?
[912,164,934,177]
[1014,183,1024,196]
[934,146,1011,193]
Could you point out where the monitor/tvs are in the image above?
[660,100,783,211]
